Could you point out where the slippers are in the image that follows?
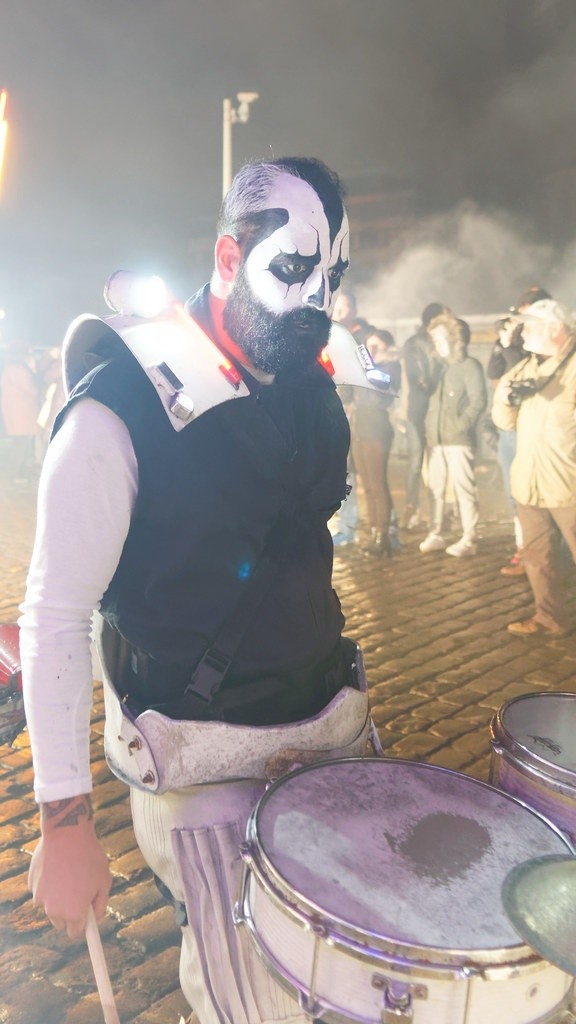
[506,616,574,637]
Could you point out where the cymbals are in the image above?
[499,850,576,980]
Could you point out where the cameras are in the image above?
[510,379,538,398]
[494,318,511,333]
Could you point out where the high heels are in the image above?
[360,527,392,558]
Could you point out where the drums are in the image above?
[239,753,576,1024]
[488,687,576,843]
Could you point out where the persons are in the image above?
[418,315,485,559]
[14,157,386,1024]
[1,286,555,576]
[489,299,576,635]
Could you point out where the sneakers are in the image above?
[419,533,447,554]
[447,540,477,557]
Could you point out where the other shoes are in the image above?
[387,522,400,550]
[329,530,356,548]
[500,551,525,575]
[397,507,417,531]
